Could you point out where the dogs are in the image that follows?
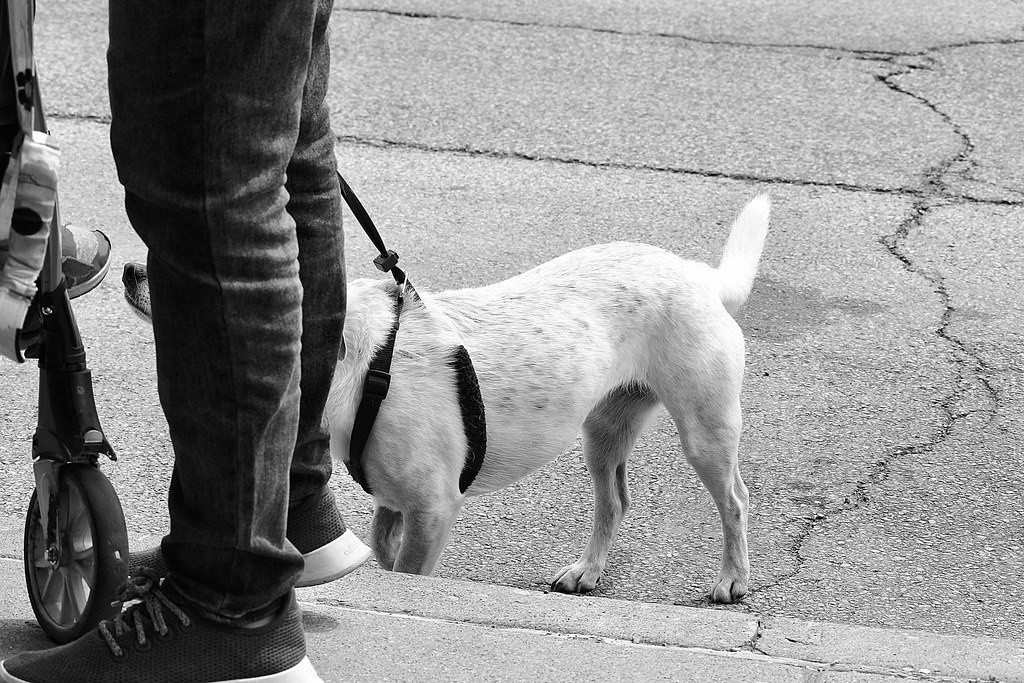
[120,189,773,604]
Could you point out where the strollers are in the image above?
[0,0,132,643]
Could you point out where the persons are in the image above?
[61,223,112,301]
[0,0,373,682]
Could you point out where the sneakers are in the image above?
[128,483,374,605]
[0,563,324,682]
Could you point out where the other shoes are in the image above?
[0,222,113,299]
